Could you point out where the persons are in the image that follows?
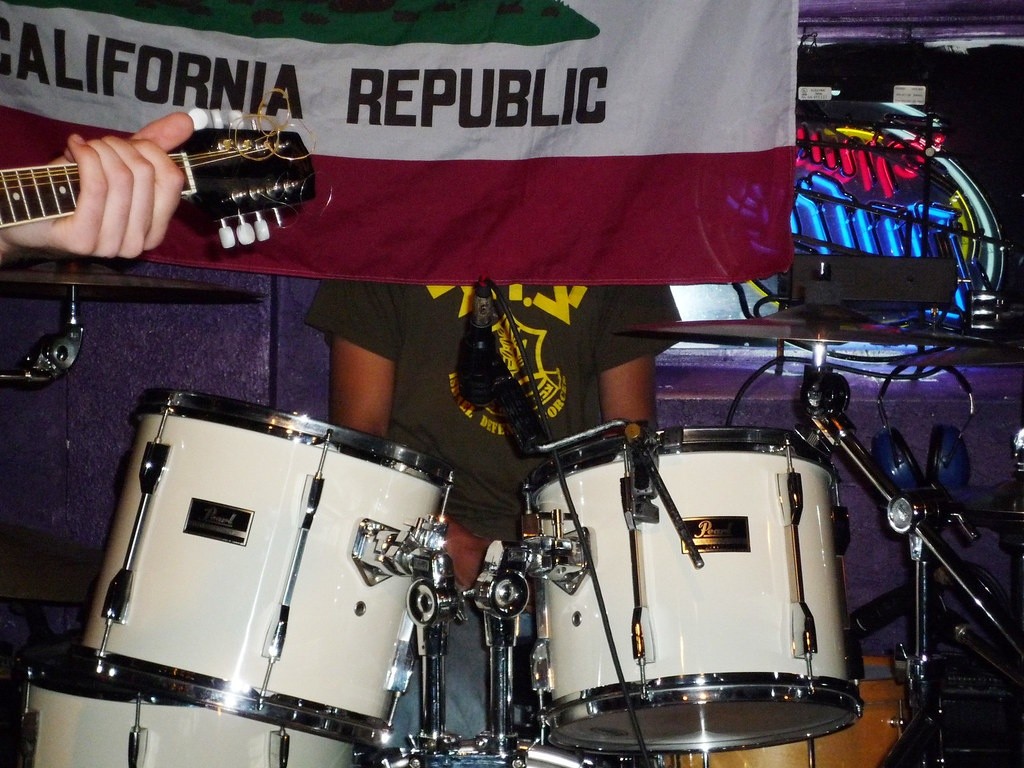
[0,112,195,266]
[307,281,684,749]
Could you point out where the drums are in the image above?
[65,382,455,730]
[649,649,923,767]
[16,660,359,768]
[527,424,867,753]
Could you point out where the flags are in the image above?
[0,0,799,284]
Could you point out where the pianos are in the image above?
[625,256,959,321]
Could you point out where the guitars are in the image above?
[0,91,320,253]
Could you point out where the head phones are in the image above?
[869,365,975,491]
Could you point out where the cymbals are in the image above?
[946,480,1024,529]
[2,267,273,300]
[0,521,113,603]
[614,300,1005,348]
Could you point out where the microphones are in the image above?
[849,586,912,640]
[455,280,499,409]
